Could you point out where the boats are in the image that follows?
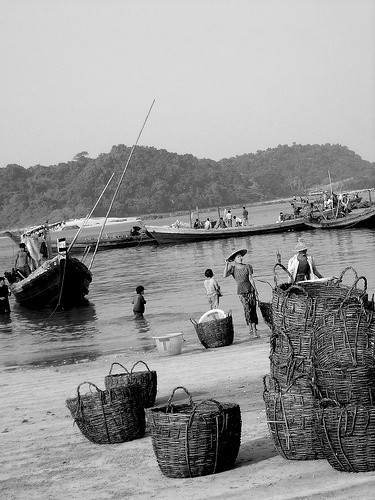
[2,96,157,314]
[137,215,312,247]
[298,189,375,230]
[3,216,145,253]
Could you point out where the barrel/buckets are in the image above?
[152,333,183,356]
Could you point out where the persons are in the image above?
[194,207,249,228]
[287,245,323,283]
[204,269,222,310]
[224,249,260,338]
[131,285,146,317]
[323,192,352,214]
[279,195,308,222]
[0,277,12,314]
[15,243,35,281]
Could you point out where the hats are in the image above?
[226,249,247,262]
[292,241,313,252]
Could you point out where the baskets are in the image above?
[146,386,243,478]
[105,361,157,410]
[263,262,375,474]
[66,379,145,444]
[190,309,234,349]
